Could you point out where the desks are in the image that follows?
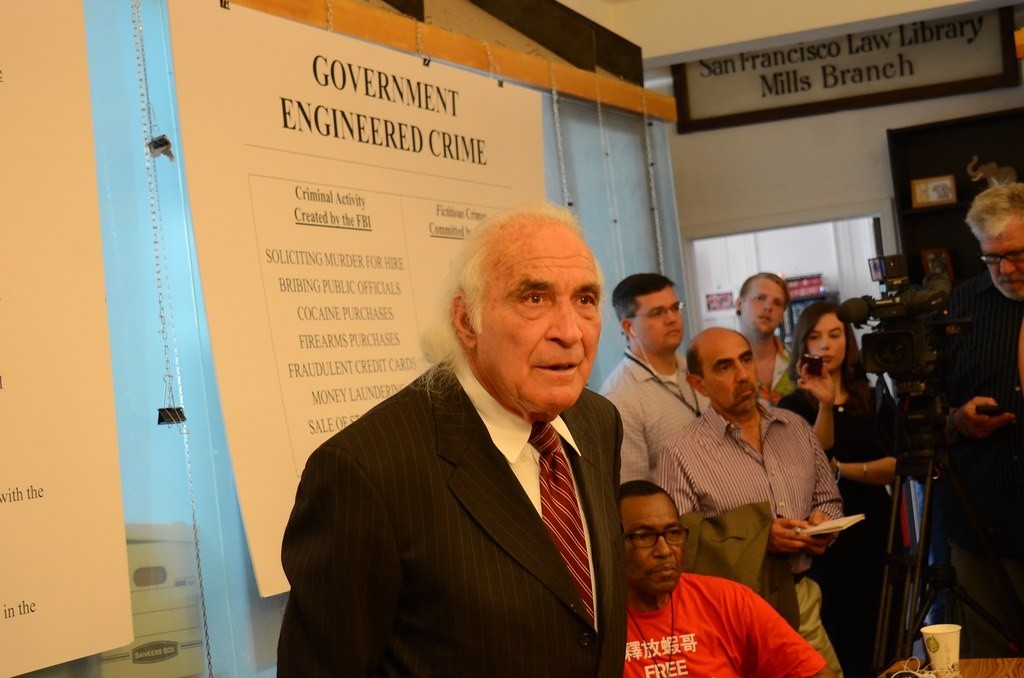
[877,657,1024,678]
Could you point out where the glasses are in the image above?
[624,526,691,548]
[626,301,683,319]
[979,250,1023,265]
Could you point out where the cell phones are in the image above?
[802,354,823,376]
[975,405,1003,417]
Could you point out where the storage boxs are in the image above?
[26,521,208,678]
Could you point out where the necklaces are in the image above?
[624,591,674,678]
[625,352,701,418]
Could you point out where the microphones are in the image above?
[836,295,877,324]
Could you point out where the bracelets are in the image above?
[949,409,961,441]
[862,463,868,483]
[834,460,841,485]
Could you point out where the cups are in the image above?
[920,624,961,678]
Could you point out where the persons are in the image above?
[735,273,797,408]
[275,207,625,678]
[598,273,709,485]
[776,302,896,678]
[616,479,838,678]
[938,183,1024,658]
[656,327,844,678]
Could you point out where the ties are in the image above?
[528,422,596,631]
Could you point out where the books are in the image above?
[793,513,865,536]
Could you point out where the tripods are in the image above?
[872,380,1024,674]
